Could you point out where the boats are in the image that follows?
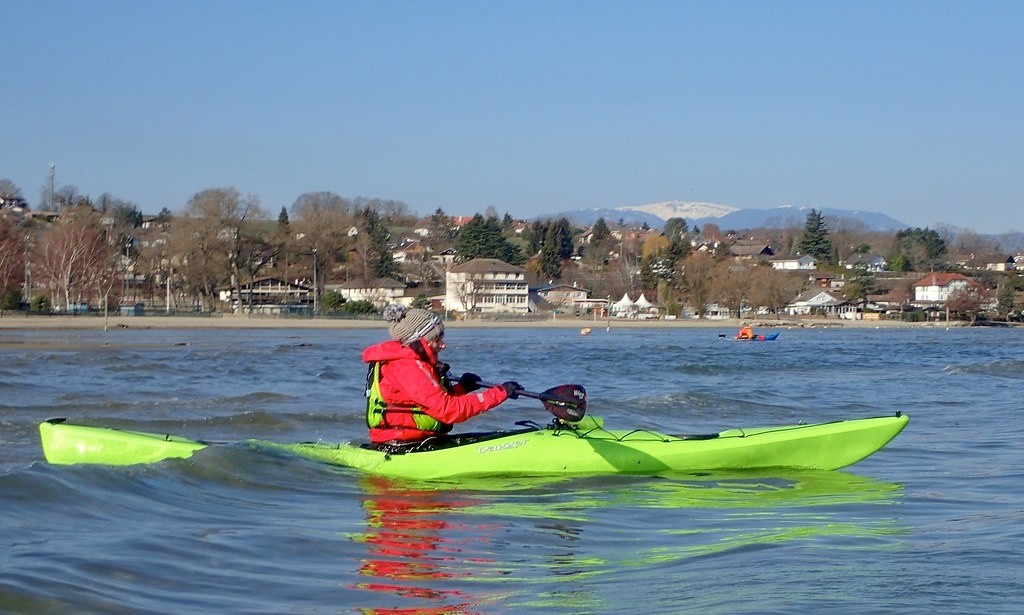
[718,331,780,340]
[39,412,912,485]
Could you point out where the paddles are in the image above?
[433,367,588,423]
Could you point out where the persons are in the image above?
[732,321,753,339]
[361,305,524,454]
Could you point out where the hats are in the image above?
[383,304,445,352]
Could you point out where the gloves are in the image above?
[459,373,482,392]
[502,381,525,399]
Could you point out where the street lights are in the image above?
[312,249,317,313]
